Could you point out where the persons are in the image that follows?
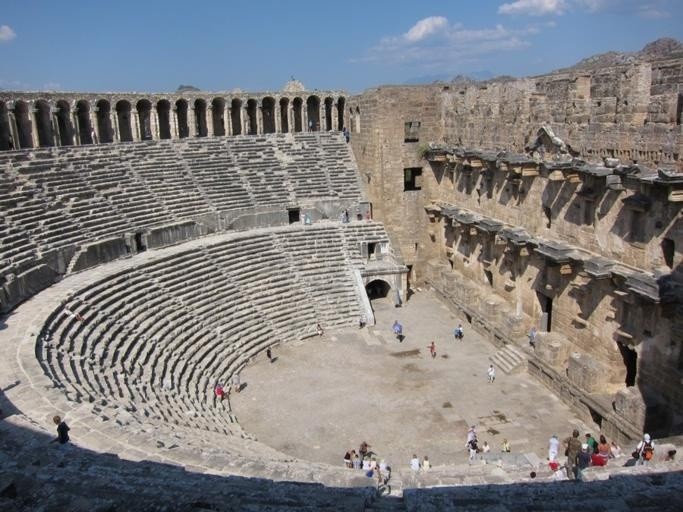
[393,321,401,341]
[344,441,389,471]
[632,434,676,465]
[265,347,272,361]
[410,455,432,471]
[425,341,436,359]
[452,325,460,341]
[340,210,345,223]
[344,209,350,223]
[47,415,70,451]
[458,325,463,343]
[214,383,223,402]
[316,324,323,335]
[500,438,511,452]
[230,371,240,392]
[465,425,489,464]
[527,326,536,348]
[485,365,494,382]
[524,429,621,481]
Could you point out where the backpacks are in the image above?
[642,441,653,460]
